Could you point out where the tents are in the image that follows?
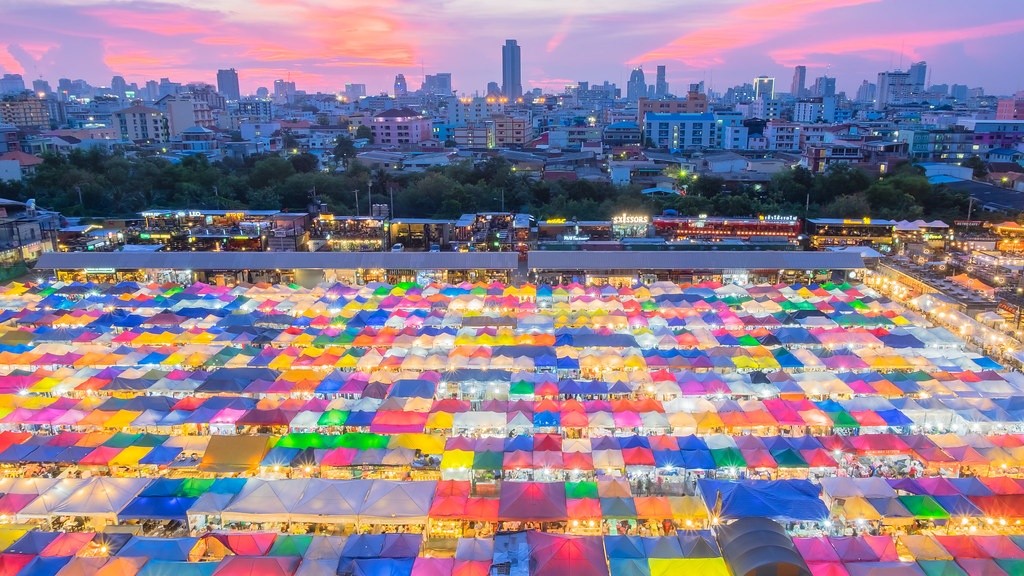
[0,221,1024,576]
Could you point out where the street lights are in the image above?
[367,180,373,217]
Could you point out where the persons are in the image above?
[60,217,1024,576]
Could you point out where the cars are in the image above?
[391,242,404,252]
[428,244,441,252]
